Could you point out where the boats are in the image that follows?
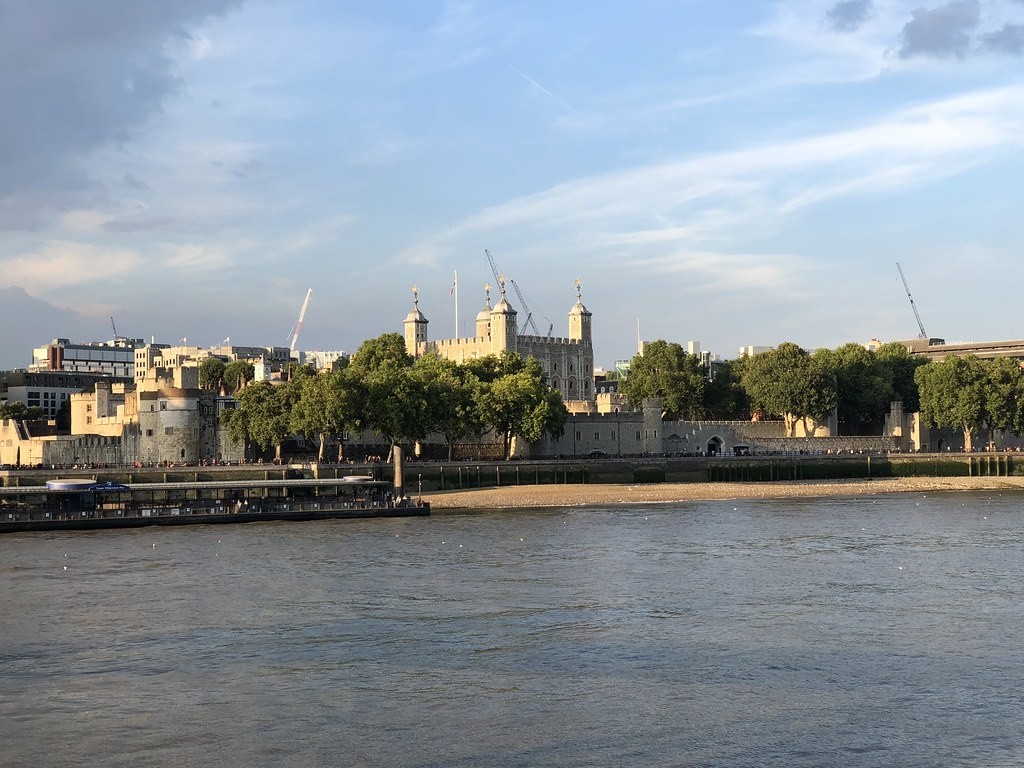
[0,476,432,535]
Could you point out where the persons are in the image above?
[11,461,109,469]
[136,458,255,466]
[385,452,531,463]
[258,455,382,464]
[383,491,417,508]
[640,445,1021,457]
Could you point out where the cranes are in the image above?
[510,279,554,337]
[896,260,928,339]
[286,287,313,351]
[484,248,508,300]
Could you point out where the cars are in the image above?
[581,449,607,459]
